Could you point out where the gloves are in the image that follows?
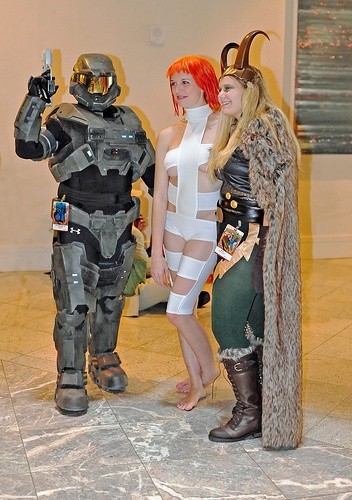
[29,69,60,104]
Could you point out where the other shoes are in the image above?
[55,369,88,411]
[90,365,128,392]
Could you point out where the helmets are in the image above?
[68,53,122,112]
[219,30,270,88]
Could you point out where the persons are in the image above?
[151,55,222,411]
[208,30,303,450]
[122,212,211,317]
[13,53,156,416]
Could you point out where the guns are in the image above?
[41,47,56,94]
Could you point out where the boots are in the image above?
[208,332,265,442]
